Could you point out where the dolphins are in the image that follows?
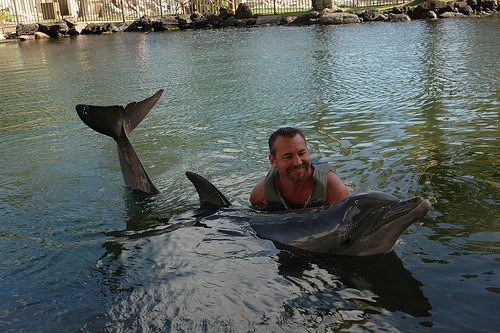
[74,88,432,259]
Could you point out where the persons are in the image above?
[249,127,350,211]
